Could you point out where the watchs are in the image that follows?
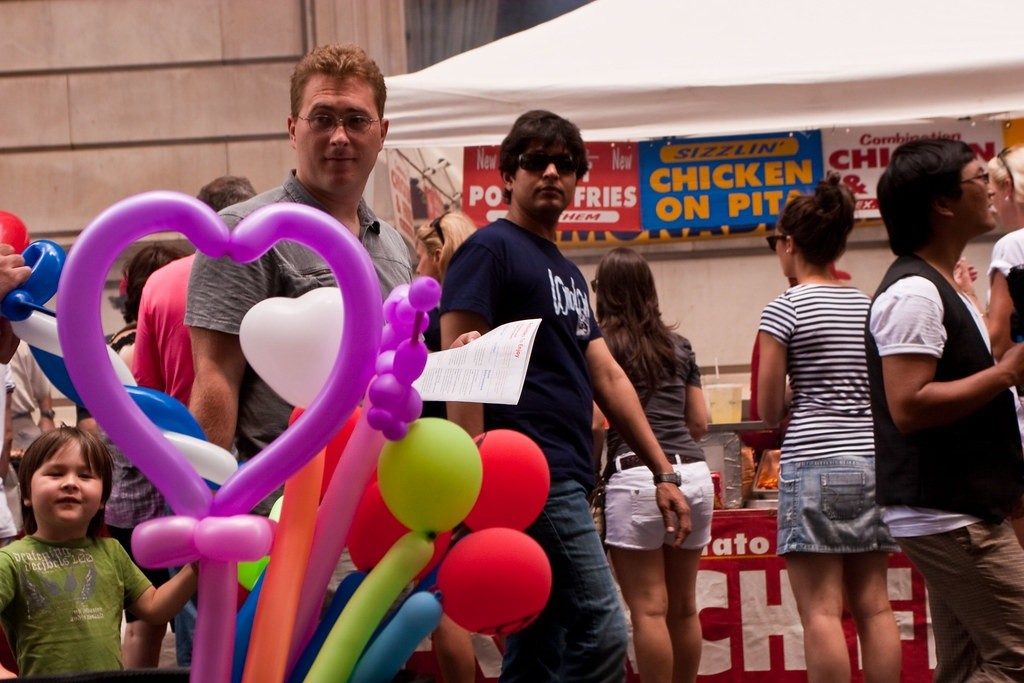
[652,472,681,487]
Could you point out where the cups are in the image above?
[706,383,743,424]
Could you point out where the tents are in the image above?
[385,0,1024,285]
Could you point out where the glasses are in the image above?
[431,209,450,246]
[516,149,578,175]
[766,233,788,251]
[294,111,381,136]
[962,171,993,189]
[591,278,599,294]
[996,143,1016,199]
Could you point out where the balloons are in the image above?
[0,191,552,683]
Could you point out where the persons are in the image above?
[0,176,255,683]
[742,138,1024,683]
[415,111,715,683]
[183,42,410,518]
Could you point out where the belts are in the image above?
[606,449,704,480]
